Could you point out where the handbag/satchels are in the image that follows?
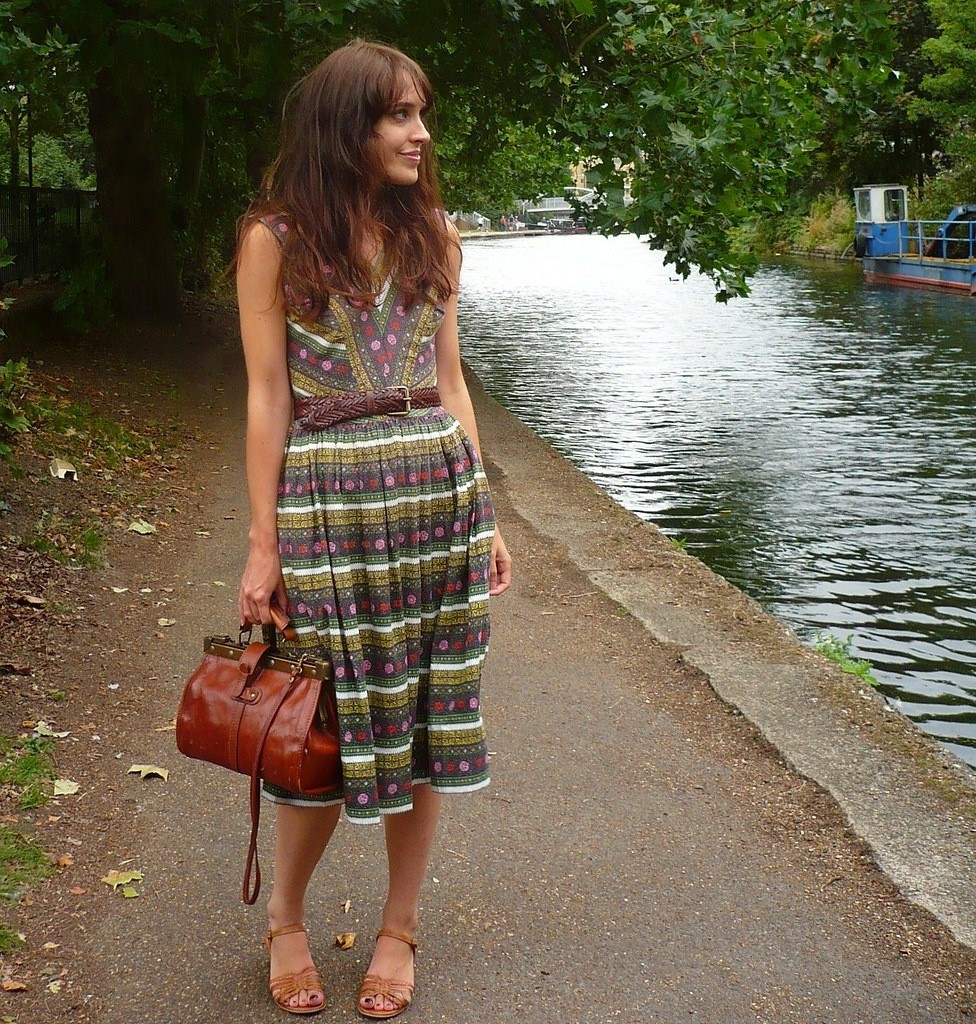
[174,605,344,796]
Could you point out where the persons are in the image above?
[500,213,519,233]
[208,40,510,1018]
[476,214,485,232]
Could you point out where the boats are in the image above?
[550,217,630,234]
[851,183,976,298]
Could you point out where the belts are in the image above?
[293,384,441,431]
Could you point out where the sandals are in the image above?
[357,928,419,1018]
[263,923,327,1014]
